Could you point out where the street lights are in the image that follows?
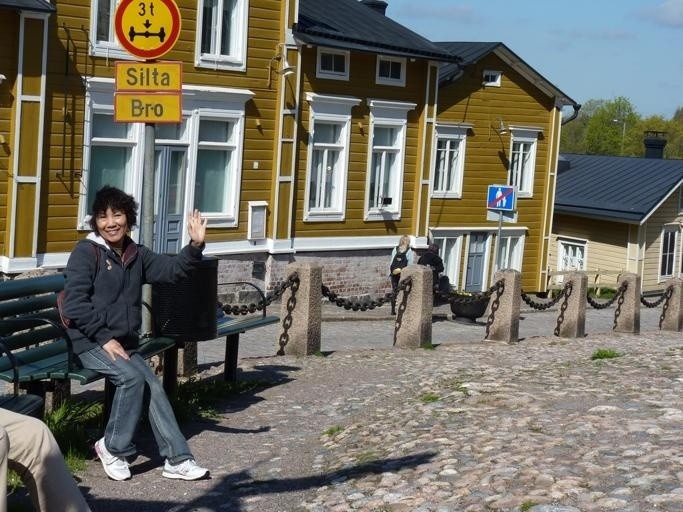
[613,119,626,156]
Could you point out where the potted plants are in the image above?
[452,290,491,323]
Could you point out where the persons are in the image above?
[1,404,96,512]
[416,242,444,307]
[57,185,211,484]
[387,233,416,316]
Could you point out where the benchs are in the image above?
[0,274,280,415]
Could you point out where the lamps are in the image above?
[488,117,508,140]
[266,52,296,87]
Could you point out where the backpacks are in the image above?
[56,237,100,330]
[389,247,411,282]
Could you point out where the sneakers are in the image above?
[93,436,132,481]
[162,458,211,481]
